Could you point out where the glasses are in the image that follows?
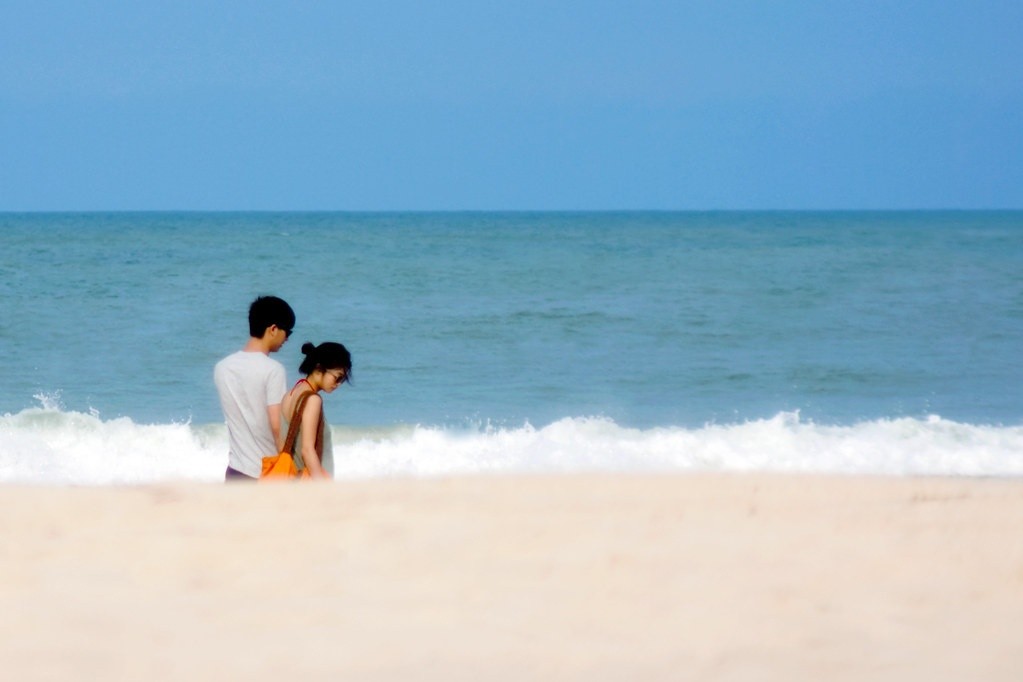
[282,326,293,338]
[326,368,346,384]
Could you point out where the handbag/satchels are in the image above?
[262,453,328,479]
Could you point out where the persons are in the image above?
[213,295,296,481]
[279,341,350,482]
